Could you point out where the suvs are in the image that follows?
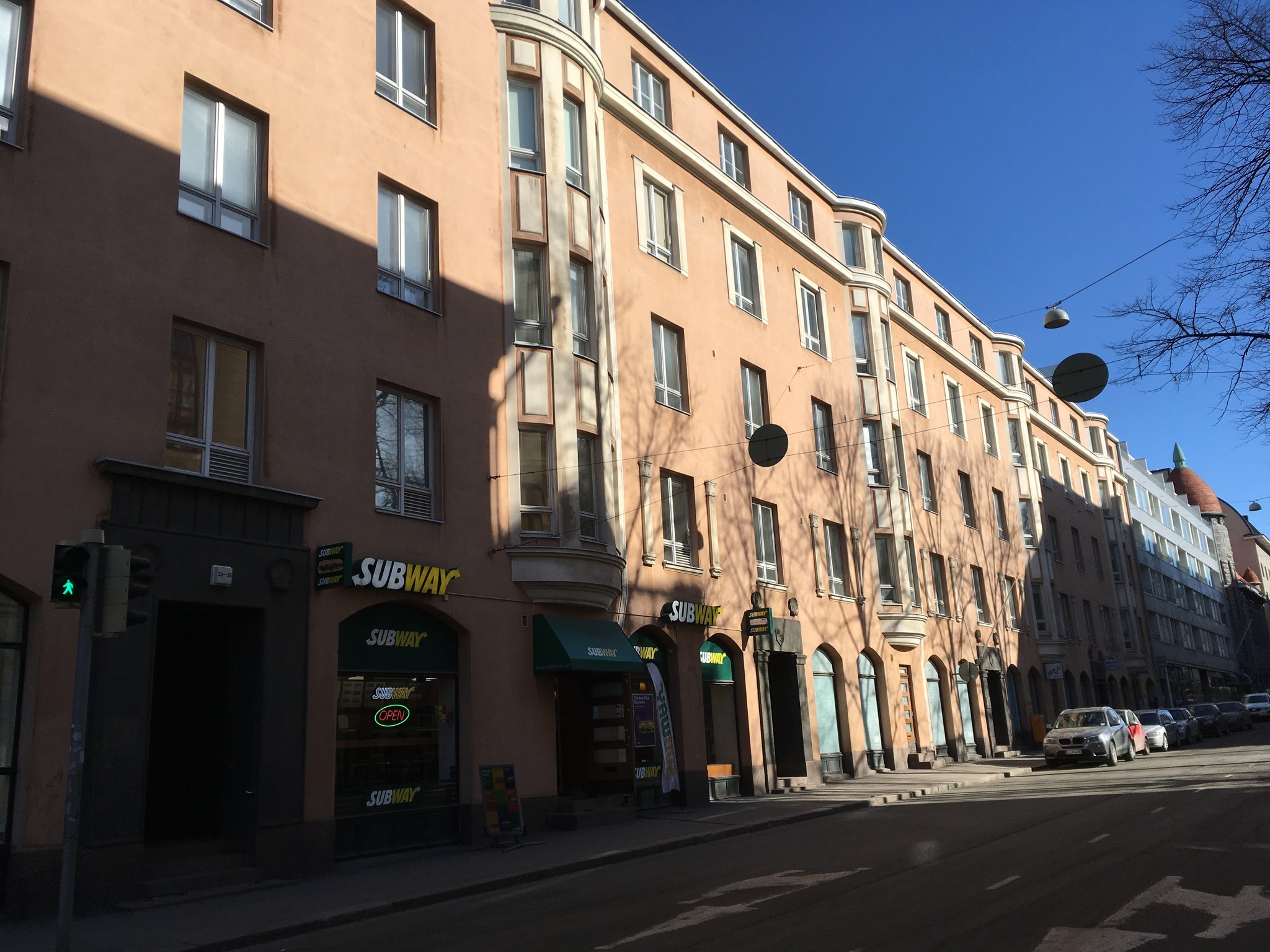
[1241,693,1270,718]
[1043,706,1135,770]
[1131,701,1253,752]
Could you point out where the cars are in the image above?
[1090,709,1150,759]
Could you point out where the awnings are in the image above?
[532,613,646,674]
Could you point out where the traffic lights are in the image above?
[49,544,82,608]
[94,545,151,633]
